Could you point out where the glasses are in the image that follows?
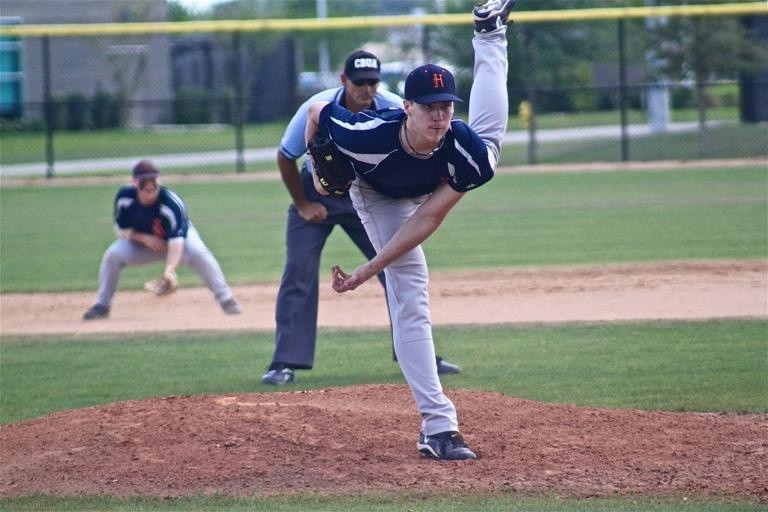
[352,79,378,86]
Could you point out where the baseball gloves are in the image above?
[143,275,173,295]
[310,135,356,194]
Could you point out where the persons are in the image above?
[259,50,464,387]
[81,161,244,320]
[304,0,518,463]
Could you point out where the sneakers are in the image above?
[472,0,516,32]
[261,362,294,385]
[435,354,461,375]
[221,297,244,314]
[84,303,111,319]
[417,430,478,460]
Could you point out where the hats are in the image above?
[404,63,463,105]
[344,50,383,82]
[133,161,161,178]
